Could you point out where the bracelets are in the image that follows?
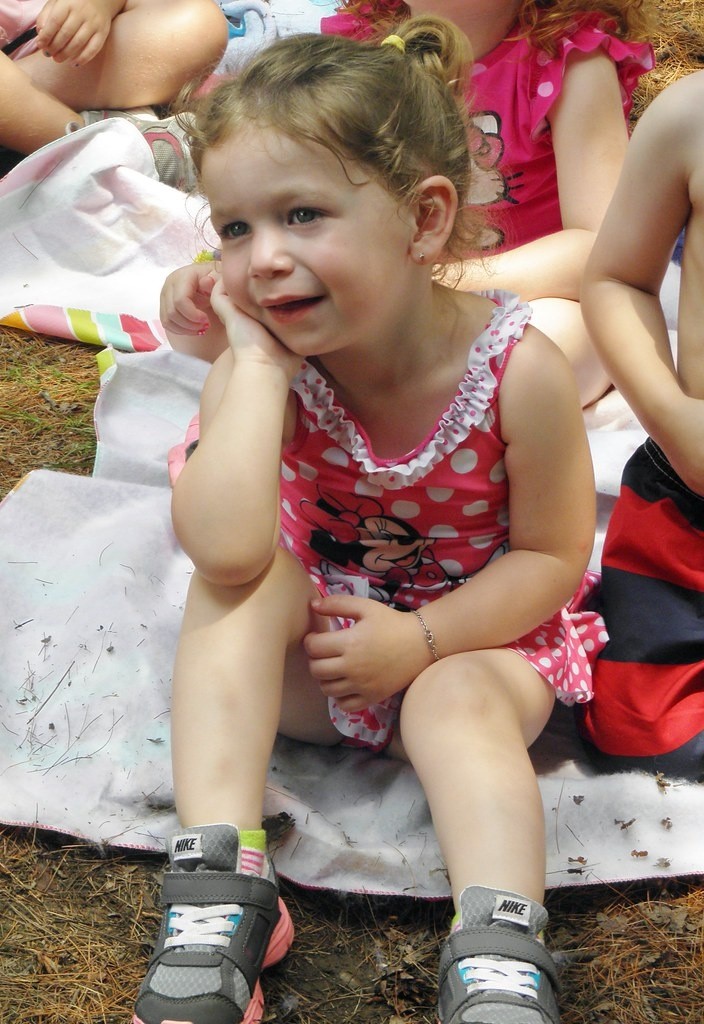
[414,610,439,661]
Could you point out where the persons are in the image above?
[0,0,228,156]
[574,69,704,783]
[336,0,655,409]
[132,16,597,1024]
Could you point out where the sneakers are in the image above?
[66,106,203,193]
[436,883,565,1024]
[131,823,294,1024]
